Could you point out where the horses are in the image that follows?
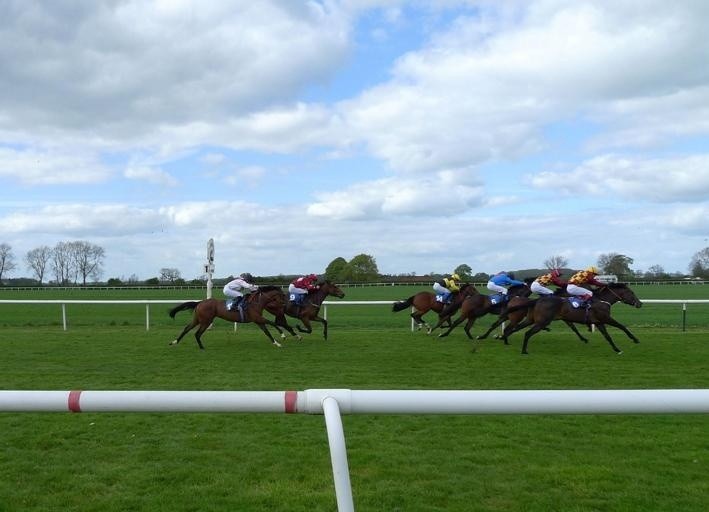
[477,278,596,344]
[263,279,344,342]
[438,275,538,340]
[168,285,288,351]
[391,282,481,335]
[498,281,643,356]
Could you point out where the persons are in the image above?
[530,270,565,295]
[289,274,319,305]
[223,275,259,310]
[487,271,528,305]
[566,267,608,308]
[433,274,461,304]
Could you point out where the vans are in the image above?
[594,274,618,284]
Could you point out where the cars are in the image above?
[681,276,705,284]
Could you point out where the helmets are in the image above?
[585,266,601,275]
[507,272,515,280]
[243,275,252,283]
[451,273,460,281]
[308,274,318,281]
[554,270,563,277]
